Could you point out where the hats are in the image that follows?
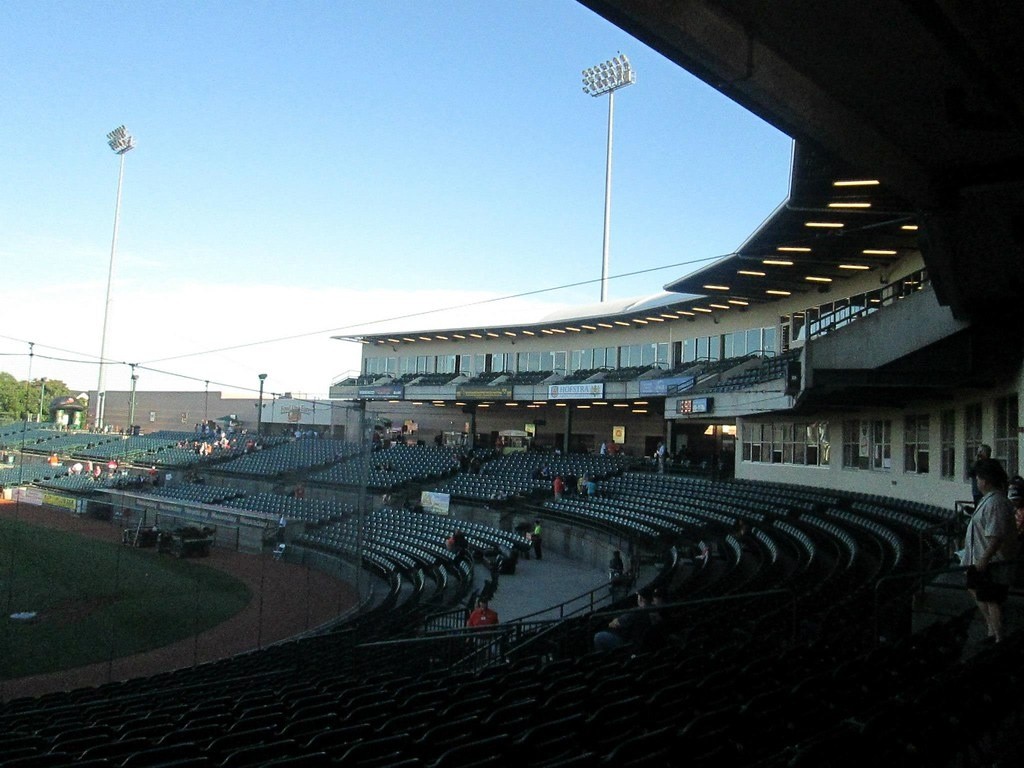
[1007,475,1024,486]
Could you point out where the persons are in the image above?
[532,463,596,497]
[149,466,157,476]
[286,428,318,440]
[214,438,231,448]
[972,444,1009,512]
[193,441,212,456]
[964,471,1017,650]
[48,452,58,465]
[1005,476,1024,543]
[275,483,305,499]
[733,519,751,540]
[195,422,225,437]
[494,436,503,459]
[466,598,497,644]
[600,440,624,457]
[72,459,117,477]
[178,439,190,449]
[593,573,683,653]
[446,529,468,551]
[657,441,666,475]
[609,550,624,586]
[278,514,287,543]
[531,520,542,561]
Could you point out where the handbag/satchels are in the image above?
[965,564,995,592]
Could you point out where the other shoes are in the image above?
[976,635,995,645]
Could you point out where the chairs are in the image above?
[351,373,802,395]
[1,413,1024,768]
